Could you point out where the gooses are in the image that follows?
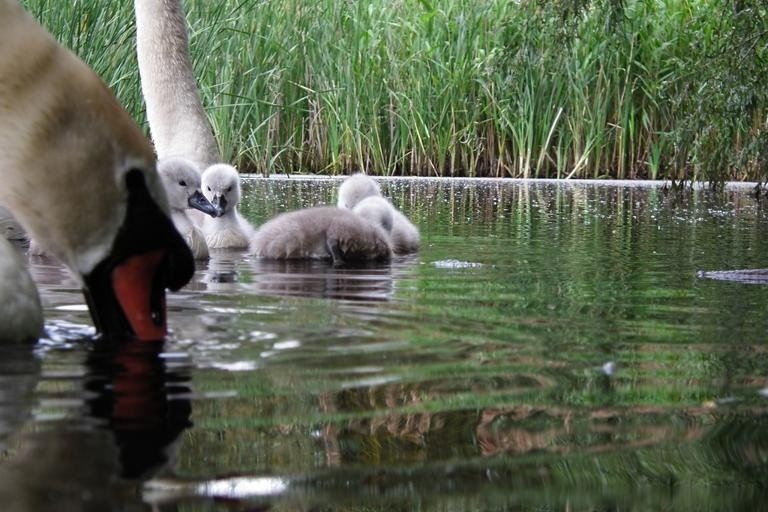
[250,172,421,271]
[22,1,252,262]
[1,4,197,346]
